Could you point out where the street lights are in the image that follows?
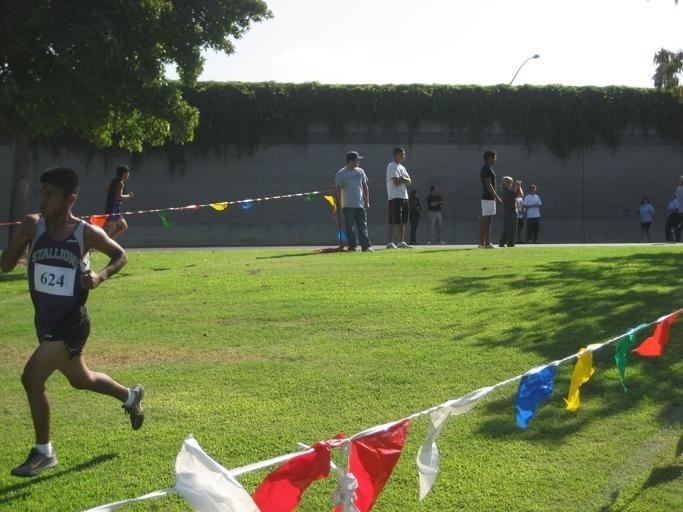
[506,52,541,86]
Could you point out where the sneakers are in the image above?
[11,448,59,476]
[121,385,145,429]
[387,242,411,249]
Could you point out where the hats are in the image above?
[347,151,364,160]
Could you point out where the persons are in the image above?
[675,175,683,231]
[479,150,503,248]
[639,193,657,242]
[665,192,680,242]
[103,164,134,241]
[333,150,375,252]
[408,190,422,245]
[386,147,413,249]
[426,184,446,245]
[514,188,525,244]
[499,175,522,247]
[522,185,543,244]
[0,164,144,478]
